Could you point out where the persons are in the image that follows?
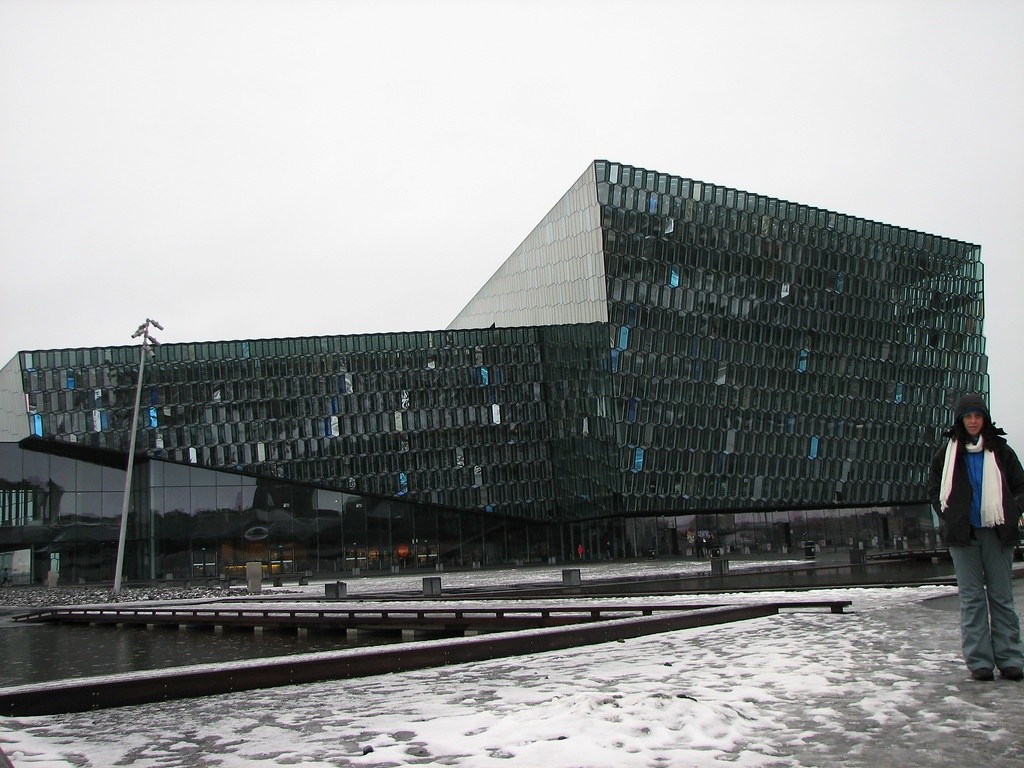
[649,534,669,547]
[693,532,714,559]
[926,395,1024,681]
[578,544,583,559]
[603,540,613,561]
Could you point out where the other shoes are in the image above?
[970,667,994,681]
[1001,666,1023,680]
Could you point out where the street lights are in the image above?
[113,318,164,592]
